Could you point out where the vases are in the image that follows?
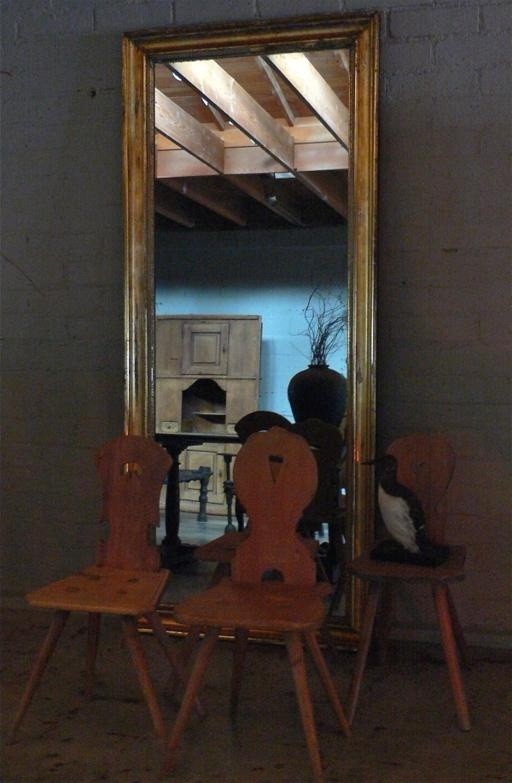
[288,364,346,428]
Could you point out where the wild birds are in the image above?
[361,456,437,564]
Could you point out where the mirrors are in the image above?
[120,8,378,653]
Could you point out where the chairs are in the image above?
[346,431,474,734]
[7,433,204,741]
[168,428,352,781]
[235,410,344,589]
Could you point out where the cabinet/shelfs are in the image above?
[155,313,262,517]
